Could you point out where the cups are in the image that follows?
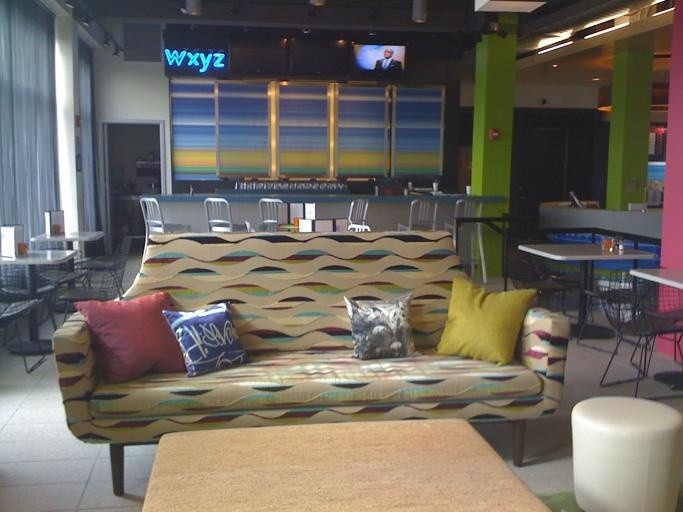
[601,236,613,253]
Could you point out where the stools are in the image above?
[571,394,682,512]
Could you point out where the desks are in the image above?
[0,246,78,357]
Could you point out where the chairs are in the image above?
[258,197,284,224]
[595,278,681,400]
[347,199,372,233]
[1,260,62,374]
[203,197,252,233]
[501,214,581,318]
[446,197,488,284]
[40,235,132,298]
[396,199,439,230]
[138,197,190,254]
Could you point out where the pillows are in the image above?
[161,301,253,377]
[72,290,186,384]
[436,276,538,367]
[342,291,415,362]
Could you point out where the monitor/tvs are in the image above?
[350,43,409,81]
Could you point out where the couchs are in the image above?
[53,230,574,498]
